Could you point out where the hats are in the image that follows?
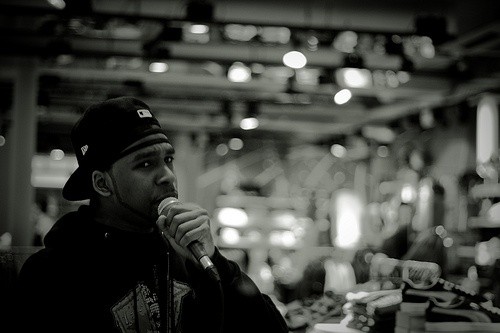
[62,96,162,204]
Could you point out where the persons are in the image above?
[1,96,292,333]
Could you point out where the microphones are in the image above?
[157,197,220,284]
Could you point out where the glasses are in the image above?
[367,253,441,292]
